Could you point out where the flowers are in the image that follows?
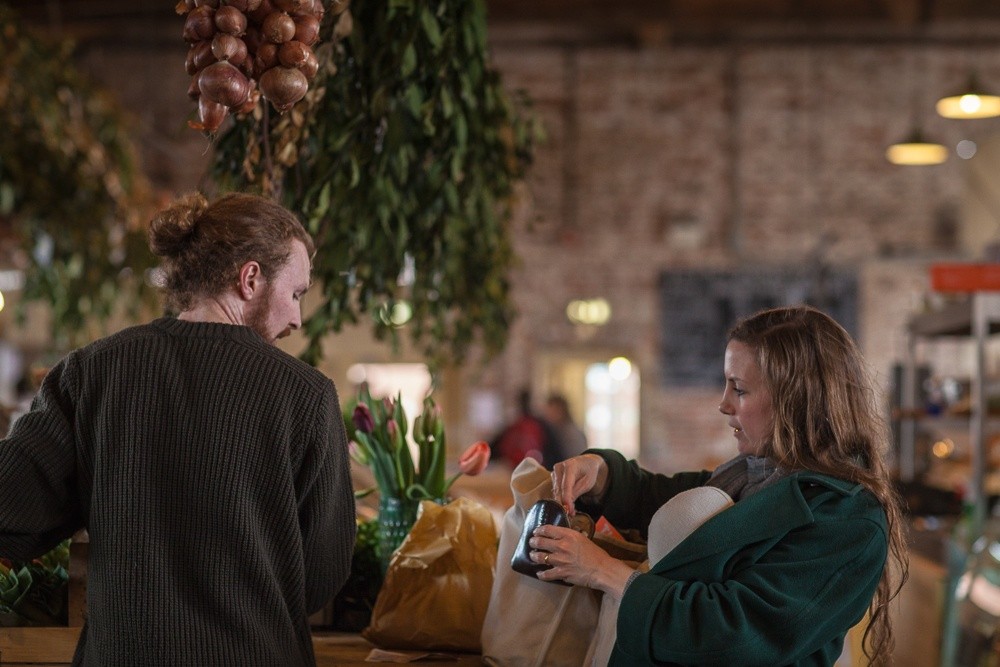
[338,377,493,496]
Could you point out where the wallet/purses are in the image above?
[511,498,595,587]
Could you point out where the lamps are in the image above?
[888,125,949,165]
[933,70,1000,120]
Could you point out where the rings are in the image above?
[544,553,549,565]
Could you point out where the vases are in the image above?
[379,498,442,579]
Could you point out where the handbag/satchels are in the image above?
[481,457,599,667]
[361,495,497,650]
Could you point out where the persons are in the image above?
[529,308,910,667]
[0,194,356,667]
[491,390,586,471]
